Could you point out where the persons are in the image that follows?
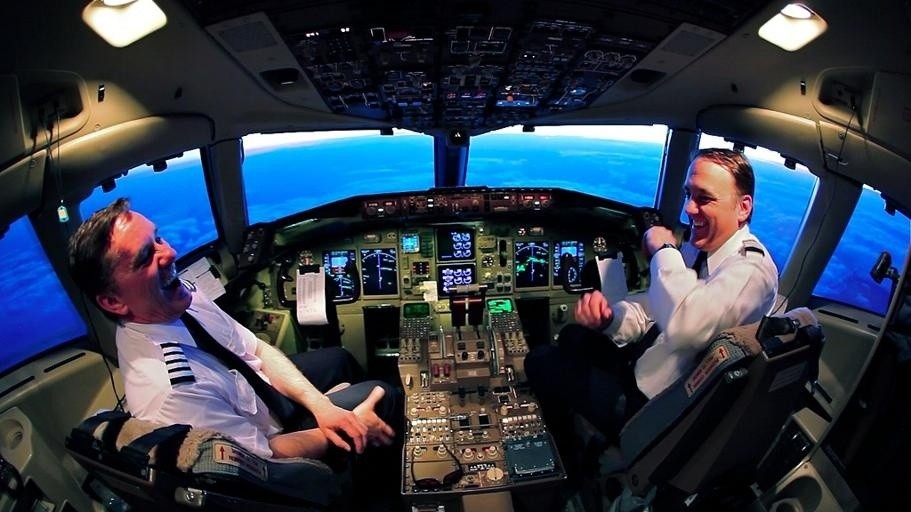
[528,148,781,434]
[63,193,405,470]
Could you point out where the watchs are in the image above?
[650,241,677,262]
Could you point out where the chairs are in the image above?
[561,306,827,512]
[68,407,366,512]
